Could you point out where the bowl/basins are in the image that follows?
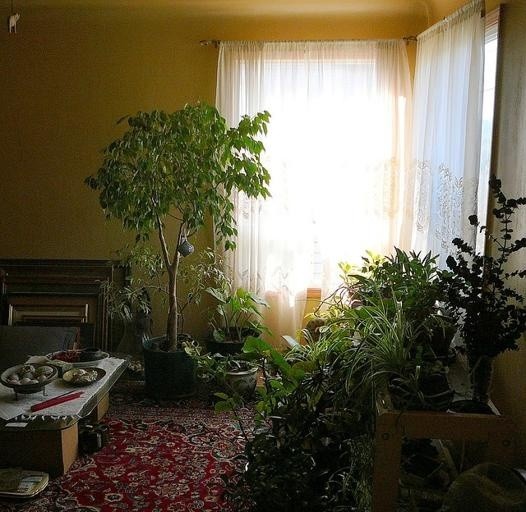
[0,363,59,392]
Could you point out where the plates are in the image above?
[63,368,106,386]
[45,350,109,369]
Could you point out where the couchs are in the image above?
[2,322,80,367]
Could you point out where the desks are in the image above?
[1,352,128,471]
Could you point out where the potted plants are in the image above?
[183,256,274,351]
[83,102,272,387]
[219,174,526,511]
[183,338,277,397]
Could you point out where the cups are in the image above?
[82,422,109,449]
